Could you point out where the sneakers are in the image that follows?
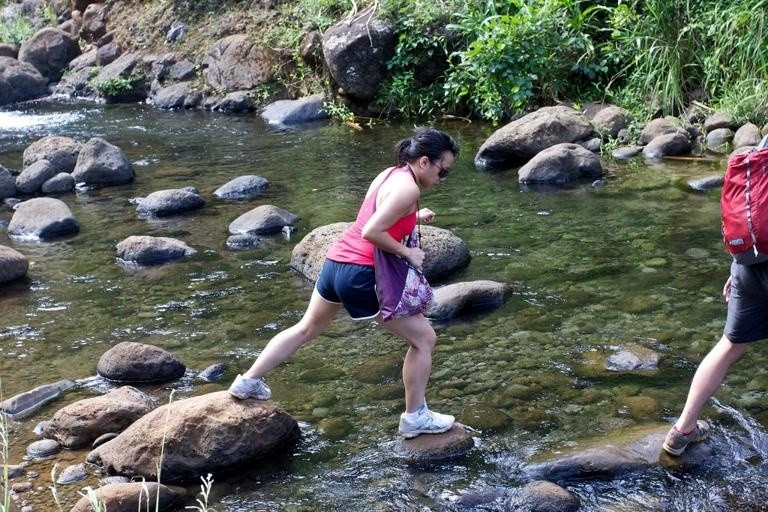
[228,374,271,400]
[399,400,455,437]
[663,420,710,455]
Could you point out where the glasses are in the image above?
[432,162,449,179]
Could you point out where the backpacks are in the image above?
[722,137,768,266]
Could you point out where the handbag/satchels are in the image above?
[374,233,437,322]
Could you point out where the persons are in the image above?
[228,131,460,441]
[662,132,767,457]
